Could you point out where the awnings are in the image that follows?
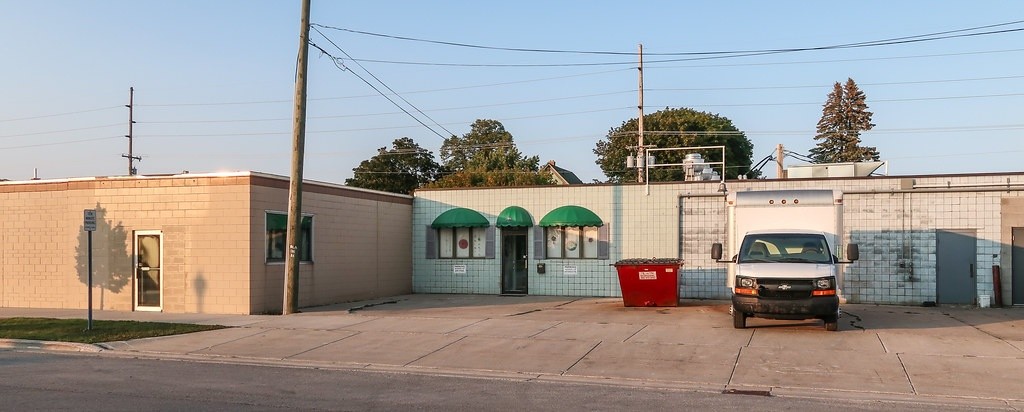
[431,208,490,229]
[539,206,603,227]
[495,206,533,227]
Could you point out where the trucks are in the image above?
[711,188,859,330]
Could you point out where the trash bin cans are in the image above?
[613,256,685,307]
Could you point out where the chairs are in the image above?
[748,242,769,259]
[801,242,822,253]
